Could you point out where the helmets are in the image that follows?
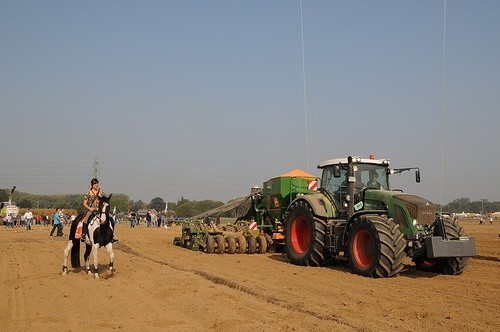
[91,178,99,184]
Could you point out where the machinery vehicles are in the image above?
[167,152,476,278]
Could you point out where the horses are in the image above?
[60,193,115,280]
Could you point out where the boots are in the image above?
[112,235,118,243]
[80,221,88,242]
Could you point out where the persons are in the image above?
[80,178,118,243]
[342,171,383,192]
[478,215,493,225]
[3,208,78,237]
[114,208,165,227]
